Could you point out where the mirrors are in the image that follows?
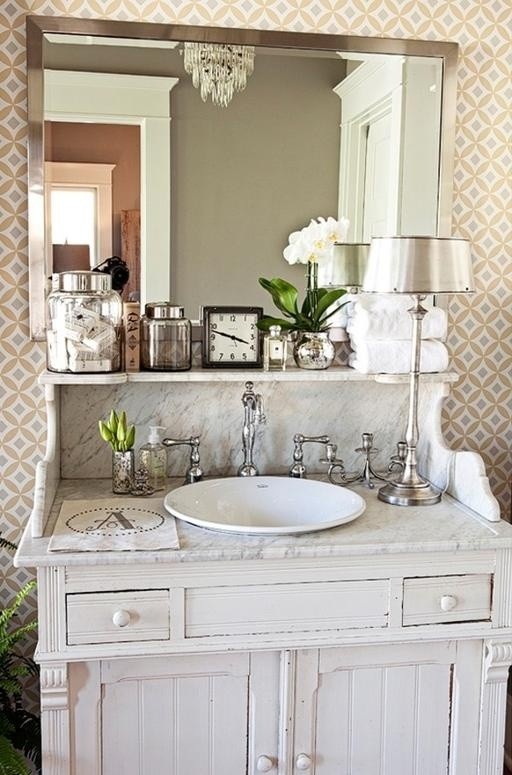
[23,12,460,344]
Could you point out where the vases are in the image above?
[293,331,335,369]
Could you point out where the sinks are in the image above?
[164,476,366,536]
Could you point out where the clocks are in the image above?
[198,305,266,370]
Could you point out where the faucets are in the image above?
[238,382,267,476]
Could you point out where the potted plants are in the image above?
[97,409,136,496]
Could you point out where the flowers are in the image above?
[255,215,352,333]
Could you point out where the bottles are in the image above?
[138,301,192,373]
[44,270,124,374]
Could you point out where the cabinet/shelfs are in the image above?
[32,548,512,775]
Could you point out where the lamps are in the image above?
[180,39,257,107]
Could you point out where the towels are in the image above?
[341,293,448,374]
